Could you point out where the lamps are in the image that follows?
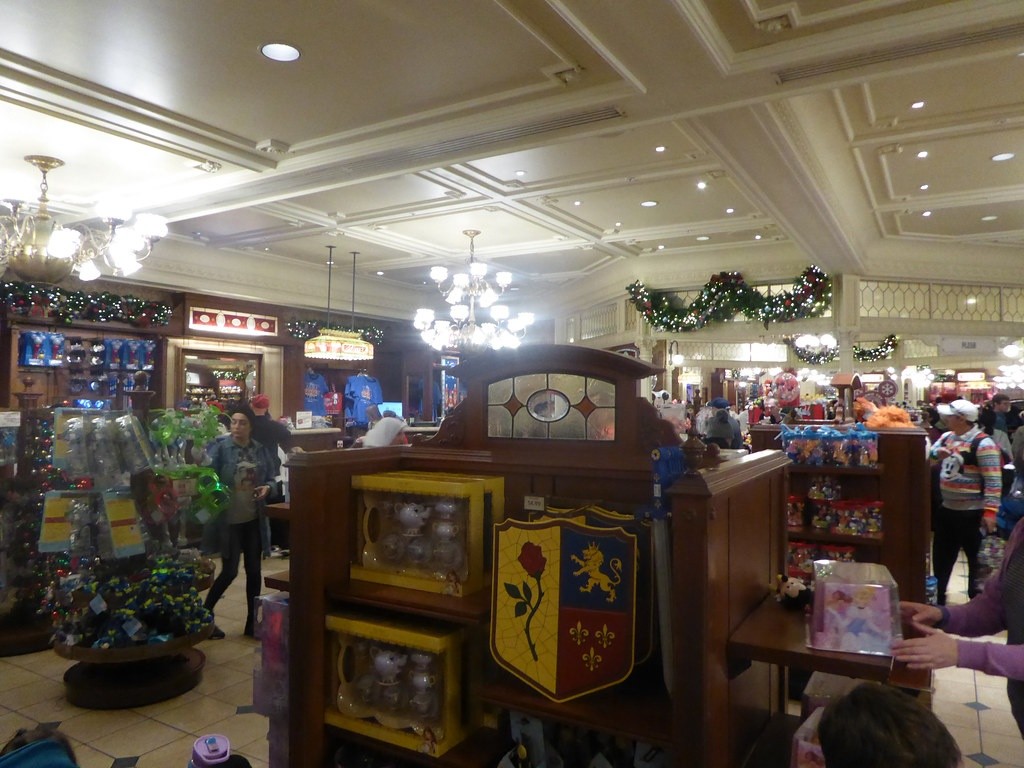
[0,155,168,282]
[304,246,374,361]
[412,231,536,350]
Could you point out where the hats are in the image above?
[253,394,270,409]
[708,398,728,408]
[705,410,734,439]
[937,399,977,422]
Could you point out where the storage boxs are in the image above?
[348,469,506,596]
[325,610,484,757]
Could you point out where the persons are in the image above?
[351,404,411,451]
[203,404,277,639]
[704,398,744,450]
[249,393,295,558]
[816,392,1024,768]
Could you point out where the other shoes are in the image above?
[244,621,255,636]
[208,626,225,640]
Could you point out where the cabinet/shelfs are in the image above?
[262,343,931,768]
